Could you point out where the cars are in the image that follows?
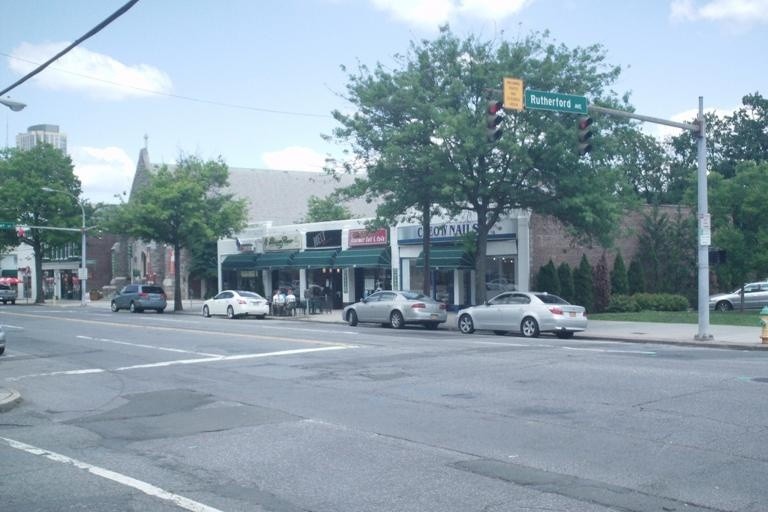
[0,327,6,355]
[709,281,768,311]
[202,290,270,319]
[0,283,16,304]
[486,278,513,290]
[111,284,167,313]
[342,291,447,329]
[292,280,323,294]
[457,292,587,338]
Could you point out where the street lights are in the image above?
[41,187,86,309]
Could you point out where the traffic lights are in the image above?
[485,99,503,146]
[576,116,592,156]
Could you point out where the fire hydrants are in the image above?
[758,304,767,347]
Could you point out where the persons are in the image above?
[305,280,331,315]
[271,288,297,317]
